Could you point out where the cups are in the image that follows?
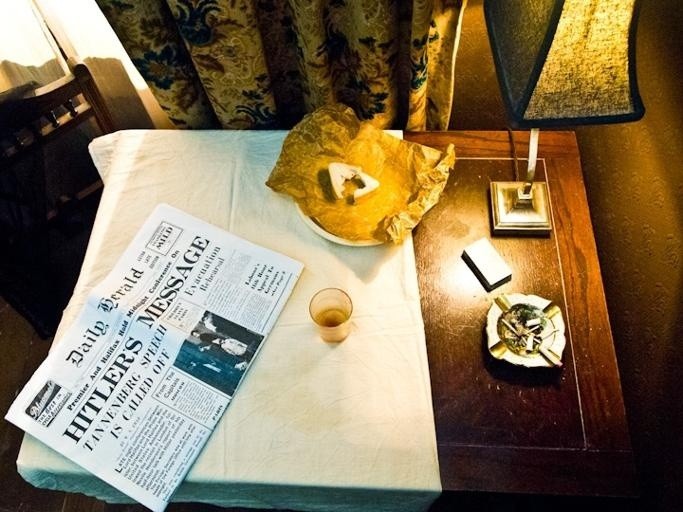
[309,287,352,344]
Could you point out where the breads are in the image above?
[326,160,381,203]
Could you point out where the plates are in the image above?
[294,200,392,248]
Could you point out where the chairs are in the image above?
[0,61,126,348]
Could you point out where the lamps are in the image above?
[475,0,648,239]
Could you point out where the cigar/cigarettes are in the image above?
[540,326,552,339]
[526,334,534,351]
[525,318,540,326]
[538,345,563,368]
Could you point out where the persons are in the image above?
[185,329,255,389]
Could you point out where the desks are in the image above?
[14,126,646,509]
